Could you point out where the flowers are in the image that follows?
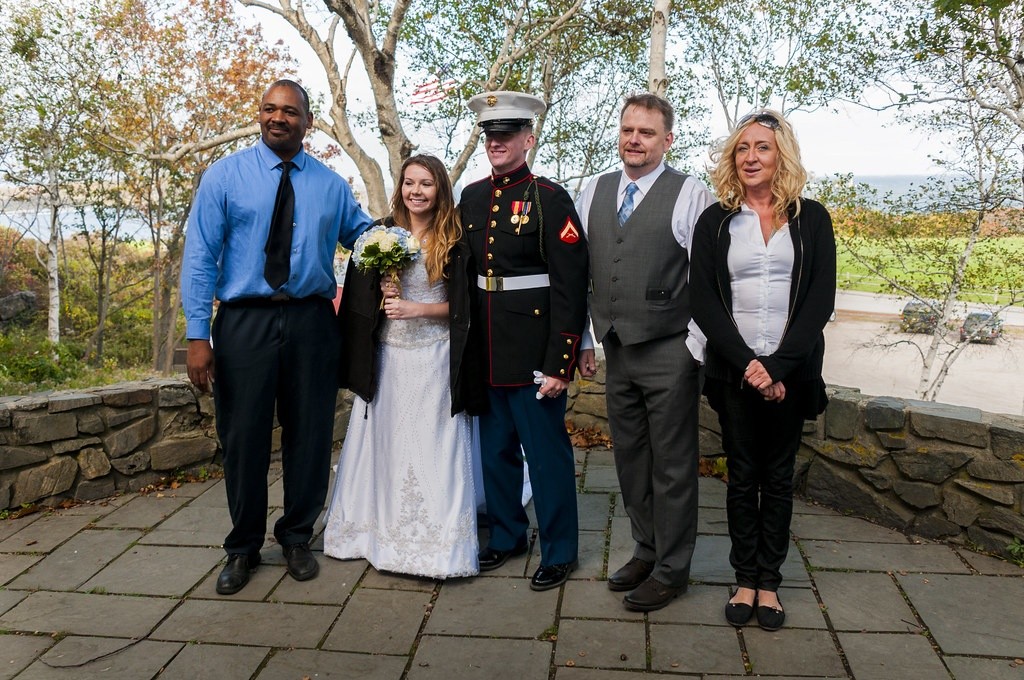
[350,225,421,309]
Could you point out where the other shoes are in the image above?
[726,582,757,625]
[757,589,785,631]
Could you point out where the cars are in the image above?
[901,301,939,333]
[961,312,1002,345]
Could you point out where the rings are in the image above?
[557,392,561,396]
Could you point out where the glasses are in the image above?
[738,114,784,136]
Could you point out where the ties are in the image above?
[264,162,297,291]
[617,182,639,228]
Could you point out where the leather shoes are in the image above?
[608,558,656,592]
[530,558,579,591]
[281,545,320,582]
[478,533,528,571]
[216,551,262,594]
[623,573,688,612]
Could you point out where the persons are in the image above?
[323,154,532,578]
[180,79,376,595]
[681,108,836,632]
[443,91,590,590]
[575,95,713,613]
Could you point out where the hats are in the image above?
[467,91,547,134]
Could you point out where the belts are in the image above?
[476,274,551,291]
